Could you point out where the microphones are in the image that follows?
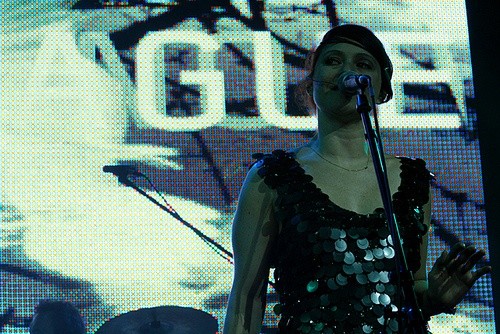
[337,70,371,96]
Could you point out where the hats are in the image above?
[314,24,393,91]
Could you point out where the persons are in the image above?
[223,25,491,334]
[29,299,86,334]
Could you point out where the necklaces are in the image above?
[307,142,370,171]
